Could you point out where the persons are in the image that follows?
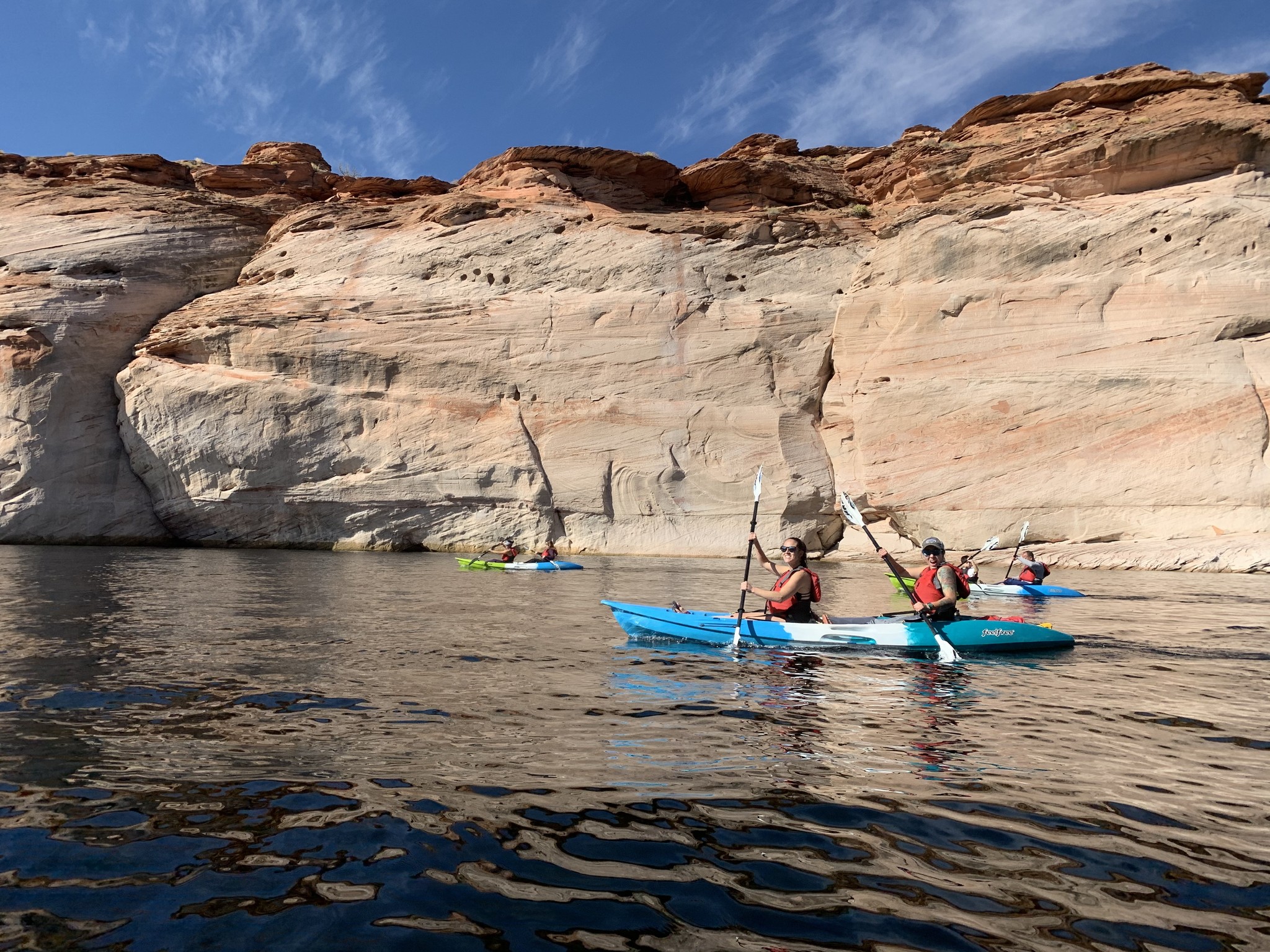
[979,551,1044,586]
[476,540,515,563]
[523,540,558,564]
[952,555,978,584]
[673,531,812,623]
[822,536,957,624]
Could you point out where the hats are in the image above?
[504,541,511,545]
[921,537,944,550]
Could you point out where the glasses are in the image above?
[922,549,943,557]
[780,546,803,553]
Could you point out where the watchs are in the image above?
[927,602,933,611]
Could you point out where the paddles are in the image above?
[840,491,960,659]
[523,545,559,568]
[1006,521,1029,578]
[469,528,521,565]
[957,536,1000,568]
[733,461,764,645]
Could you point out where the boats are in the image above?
[604,601,1074,652]
[455,557,584,570]
[885,572,1084,598]
